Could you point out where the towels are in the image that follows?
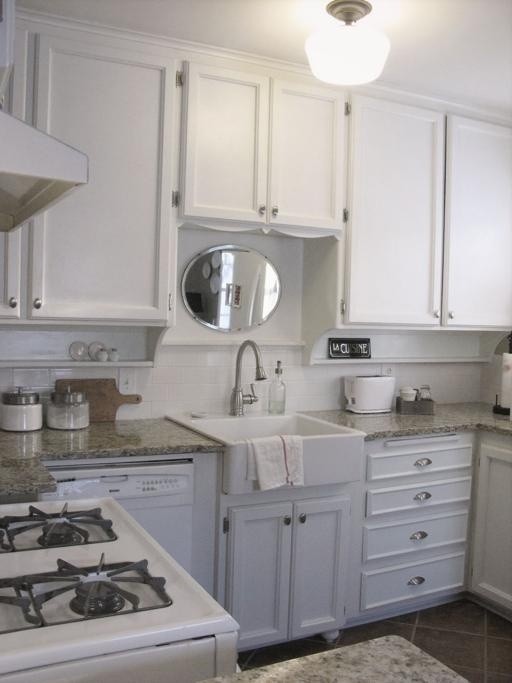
[244,434,305,492]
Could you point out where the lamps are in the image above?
[305,0,391,86]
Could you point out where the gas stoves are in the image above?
[0,495,241,674]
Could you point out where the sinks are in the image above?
[172,412,365,495]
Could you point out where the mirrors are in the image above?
[181,244,282,333]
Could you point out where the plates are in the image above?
[69,342,88,361]
[211,250,221,269]
[201,262,212,280]
[88,342,105,361]
[209,272,221,295]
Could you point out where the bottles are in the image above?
[267,360,287,416]
[417,384,431,401]
[109,348,120,362]
[96,349,108,361]
[0,385,43,433]
[47,384,90,430]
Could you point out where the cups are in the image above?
[399,386,417,401]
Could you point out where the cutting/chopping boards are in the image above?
[54,379,143,424]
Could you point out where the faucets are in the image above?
[231,338,267,416]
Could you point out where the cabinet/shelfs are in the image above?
[177,39,345,242]
[301,79,512,365]
[213,482,352,654]
[339,429,512,630]
[0,7,171,369]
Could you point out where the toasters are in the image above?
[342,375,396,415]
